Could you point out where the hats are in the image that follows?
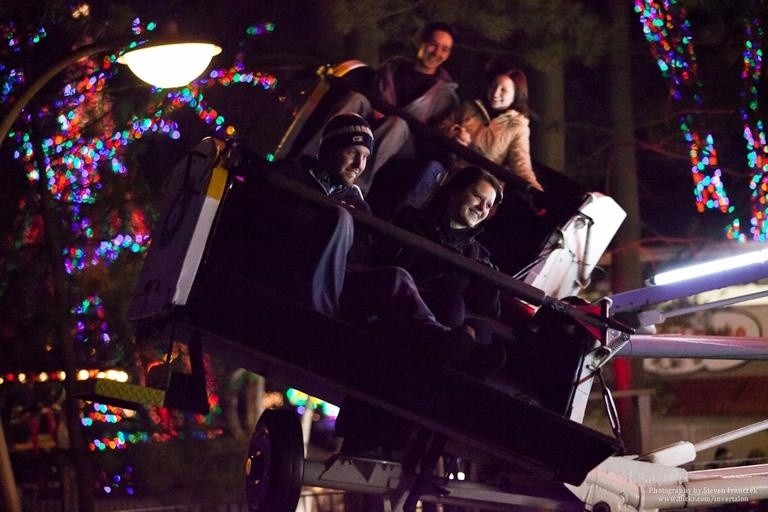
[317,109,375,160]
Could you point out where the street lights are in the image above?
[0,34,226,146]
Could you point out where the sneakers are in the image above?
[405,321,508,381]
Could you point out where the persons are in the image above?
[400,164,502,347]
[271,21,459,220]
[391,61,544,221]
[704,447,764,512]
[246,112,452,350]
